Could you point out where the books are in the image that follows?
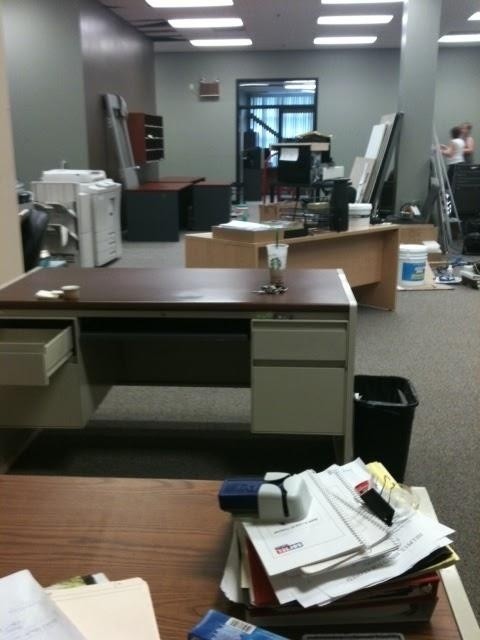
[0,568,162,640]
[223,470,461,631]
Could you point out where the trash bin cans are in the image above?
[353,373,420,484]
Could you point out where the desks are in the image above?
[0,475,480,638]
[125,182,195,244]
[148,173,205,220]
[194,181,235,229]
[0,267,361,466]
[185,215,401,321]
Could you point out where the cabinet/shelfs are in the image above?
[125,112,167,165]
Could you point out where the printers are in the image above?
[29,167,124,269]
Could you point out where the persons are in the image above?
[439,127,466,189]
[438,121,475,165]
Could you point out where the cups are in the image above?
[267,243,289,289]
